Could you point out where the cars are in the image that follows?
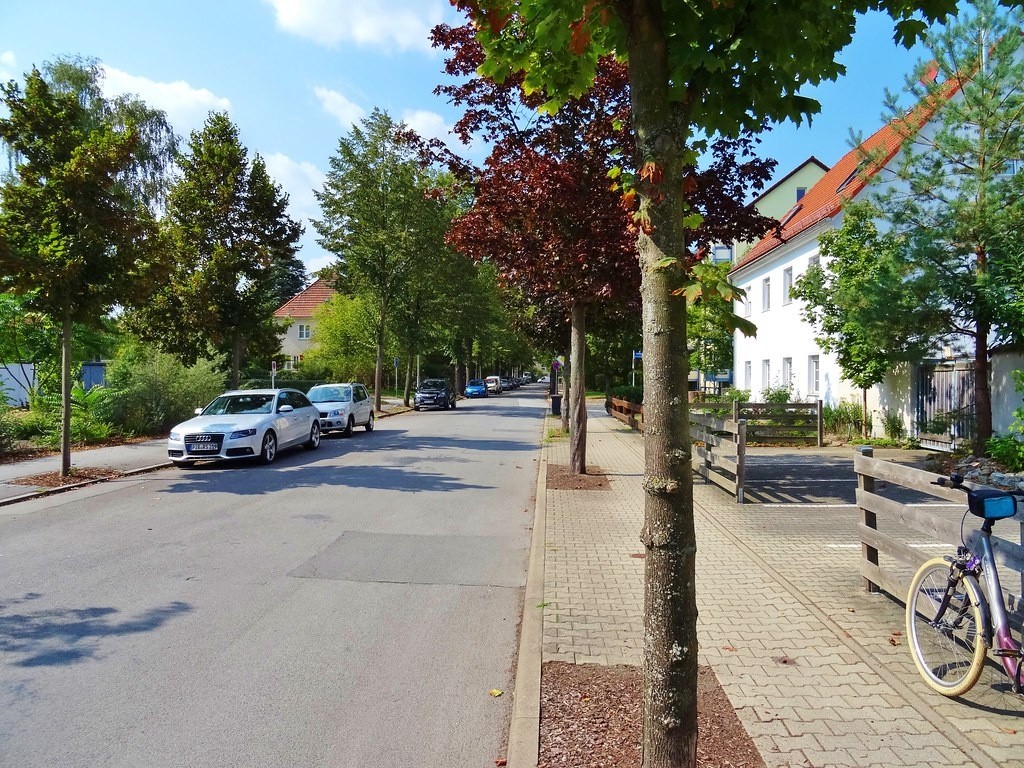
[465,379,488,399]
[304,383,374,437]
[414,379,456,411]
[484,371,532,394]
[168,388,321,468]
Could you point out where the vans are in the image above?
[537,375,550,384]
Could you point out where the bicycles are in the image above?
[905,474,1024,696]
